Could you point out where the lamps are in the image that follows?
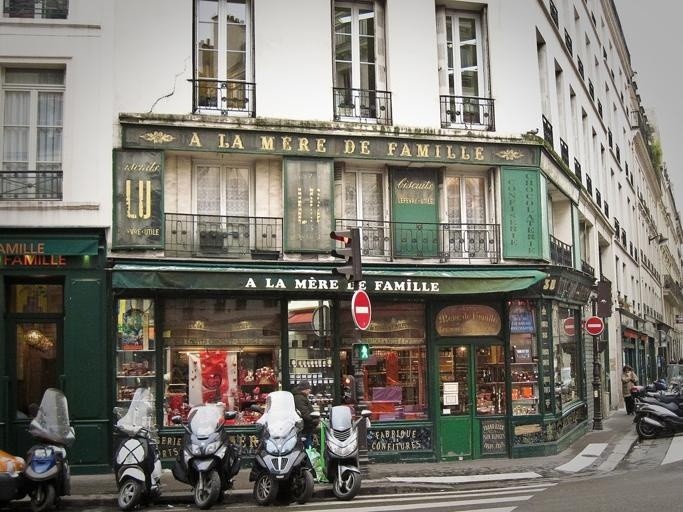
[647,233,669,246]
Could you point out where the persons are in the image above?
[294,380,320,448]
[621,365,639,416]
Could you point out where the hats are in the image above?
[298,381,311,390]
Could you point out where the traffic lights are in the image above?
[352,343,370,361]
[330,227,363,283]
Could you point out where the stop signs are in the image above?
[563,316,576,336]
[351,290,372,331]
[585,316,605,336]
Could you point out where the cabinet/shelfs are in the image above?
[364,347,454,389]
[485,362,538,386]
[114,348,157,403]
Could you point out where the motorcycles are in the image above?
[309,405,373,501]
[630,379,683,440]
[249,390,317,507]
[171,404,242,509]
[112,387,163,511]
[25,387,77,512]
